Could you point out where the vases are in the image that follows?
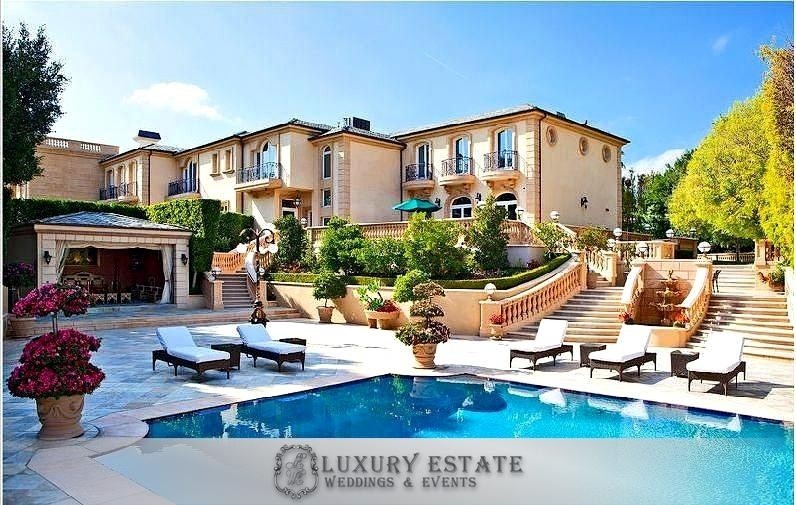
[10,318,36,339]
[35,394,85,441]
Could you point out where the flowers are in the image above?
[4,263,33,305]
[7,284,104,398]
[12,298,31,317]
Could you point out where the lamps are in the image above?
[182,254,188,265]
[44,251,51,264]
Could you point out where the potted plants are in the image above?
[362,281,398,330]
[396,281,450,368]
[314,275,347,323]
[395,271,427,308]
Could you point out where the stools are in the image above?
[90,293,104,305]
[106,293,117,303]
[120,293,132,303]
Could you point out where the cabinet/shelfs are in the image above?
[580,344,606,368]
[211,343,239,372]
[670,351,698,378]
[279,338,306,363]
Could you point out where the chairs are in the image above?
[132,276,155,300]
[686,333,746,395]
[510,319,573,371]
[589,325,656,382]
[145,281,167,303]
[232,324,306,372]
[152,327,231,383]
[61,272,105,294]
